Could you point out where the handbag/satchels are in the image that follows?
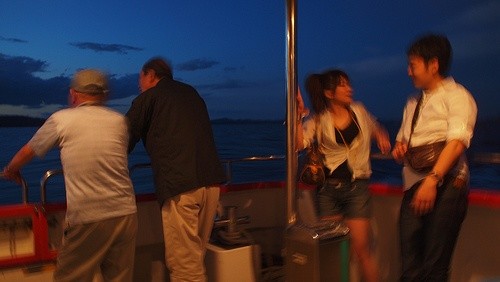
[404,140,458,171]
[301,114,325,184]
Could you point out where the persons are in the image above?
[125,57,226,282]
[391,33,477,282]
[2,70,138,282]
[296,69,392,282]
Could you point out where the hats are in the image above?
[71,70,113,100]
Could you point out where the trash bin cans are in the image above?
[285,221,352,282]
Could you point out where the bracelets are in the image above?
[428,171,445,183]
